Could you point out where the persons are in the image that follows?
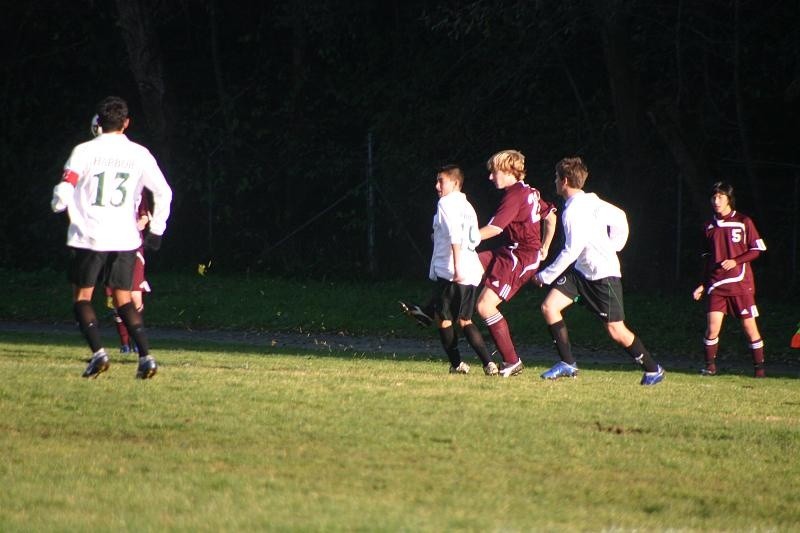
[690,174,768,378]
[427,164,500,376]
[526,157,668,386]
[103,184,150,354]
[49,101,171,379]
[398,149,556,375]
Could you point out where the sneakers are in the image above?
[120,345,131,354]
[499,357,525,377]
[482,363,498,375]
[396,299,435,328]
[135,360,157,379]
[82,356,109,377]
[540,360,579,379]
[640,363,665,385]
[450,360,470,375]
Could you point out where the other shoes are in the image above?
[755,367,765,378]
[701,367,716,376]
